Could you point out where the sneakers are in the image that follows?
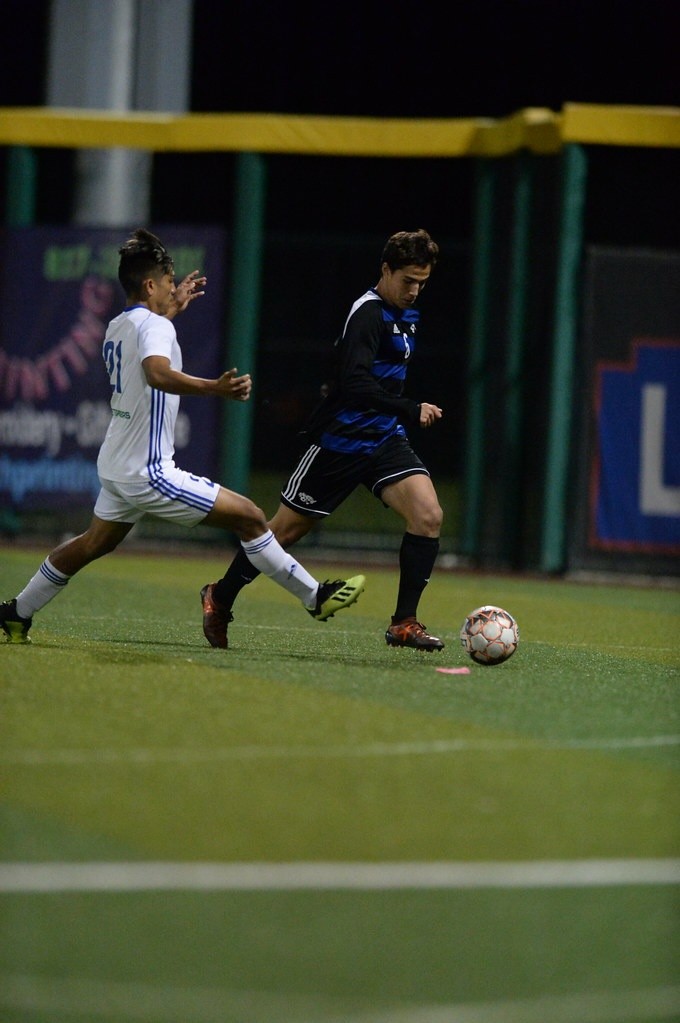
[0,598,33,643]
[385,616,444,653]
[304,575,366,623]
[201,579,234,650]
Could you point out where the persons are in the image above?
[198,229,445,652]
[0,227,366,644]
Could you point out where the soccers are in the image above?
[461,604,518,663]
